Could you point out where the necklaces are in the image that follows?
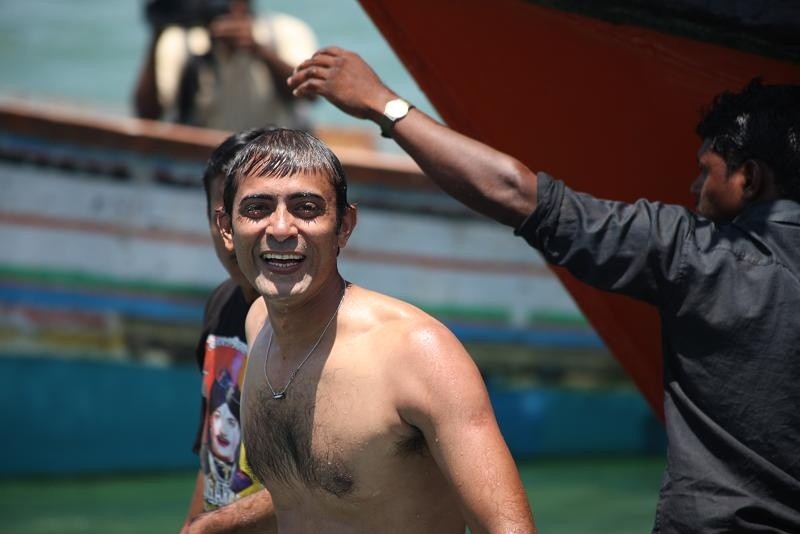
[264,282,346,400]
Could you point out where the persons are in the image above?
[179,125,279,534]
[134,0,318,131]
[222,126,539,534]
[287,47,800,534]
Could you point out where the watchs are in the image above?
[381,98,415,138]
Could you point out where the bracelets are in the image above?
[253,43,262,61]
